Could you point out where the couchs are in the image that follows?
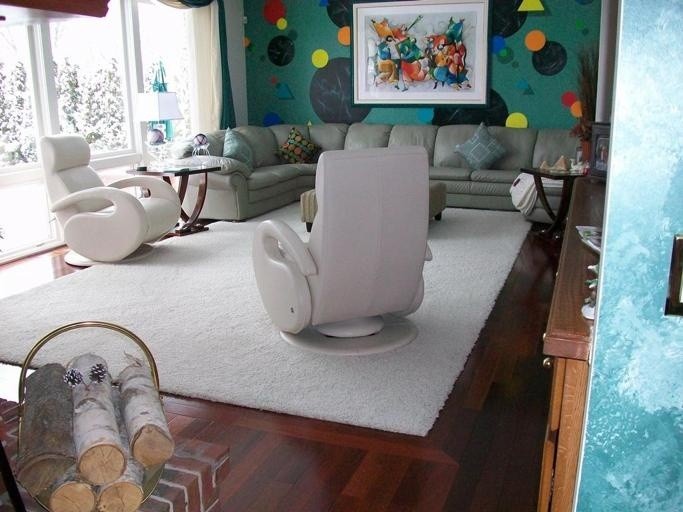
[174,121,581,220]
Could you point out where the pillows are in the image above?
[453,121,506,171]
[276,126,322,163]
[223,126,254,172]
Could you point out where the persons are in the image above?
[594,143,608,172]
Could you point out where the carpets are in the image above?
[0,201,532,438]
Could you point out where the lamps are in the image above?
[135,91,183,145]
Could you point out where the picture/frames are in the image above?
[353,1,488,105]
[591,123,610,177]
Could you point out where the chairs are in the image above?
[252,148,432,357]
[42,133,181,267]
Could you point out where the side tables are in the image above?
[127,166,222,236]
[520,169,583,241]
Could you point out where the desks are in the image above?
[537,176,606,512]
